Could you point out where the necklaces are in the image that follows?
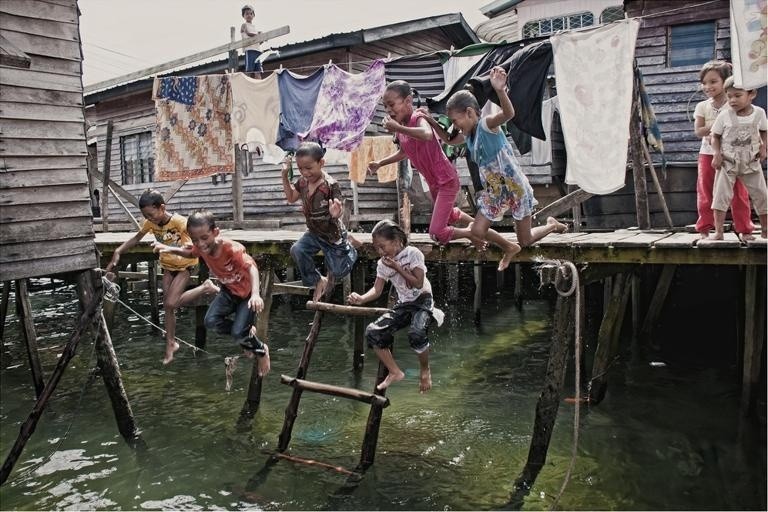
[710,94,728,113]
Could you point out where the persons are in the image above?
[149,209,272,379]
[692,60,757,242]
[239,3,264,79]
[280,140,367,303]
[107,187,223,363]
[706,76,767,241]
[348,219,434,391]
[415,65,569,271]
[367,79,490,253]
